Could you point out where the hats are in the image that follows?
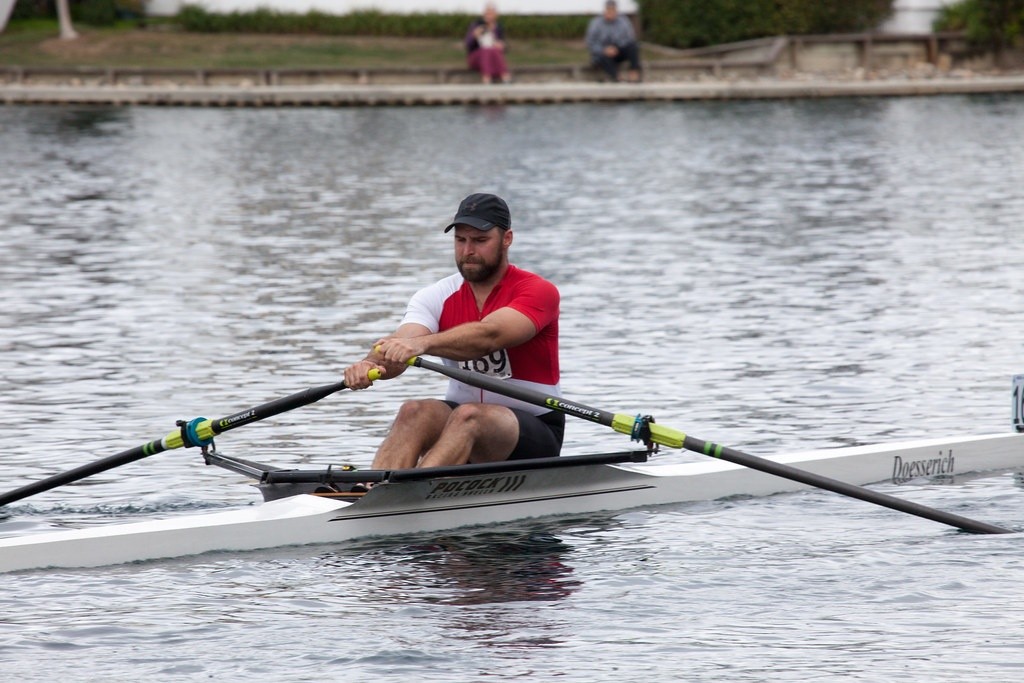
[444,193,511,231]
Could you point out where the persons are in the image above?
[318,194,566,495]
[584,1,643,83]
[466,4,512,85]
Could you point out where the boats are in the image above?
[0,375,1024,573]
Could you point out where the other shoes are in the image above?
[350,480,375,493]
[314,483,342,494]
